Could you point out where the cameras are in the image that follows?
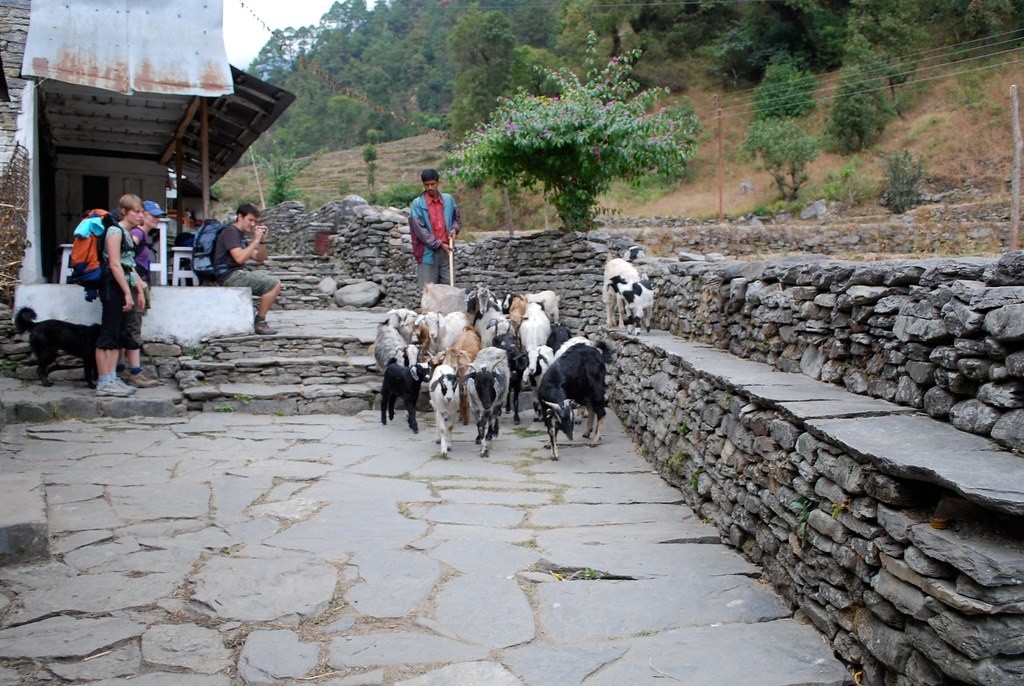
[261,226,267,234]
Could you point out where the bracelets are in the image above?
[259,241,268,244]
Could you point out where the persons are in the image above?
[210,203,279,335]
[407,168,462,304]
[94,193,147,397]
[116,200,167,388]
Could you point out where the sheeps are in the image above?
[532,336,612,460]
[600,258,661,337]
[374,282,563,459]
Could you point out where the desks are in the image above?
[145,218,170,286]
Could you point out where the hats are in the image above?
[143,201,168,216]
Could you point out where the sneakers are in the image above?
[129,371,158,387]
[96,381,131,397]
[117,367,132,381]
[256,321,278,334]
[115,378,137,393]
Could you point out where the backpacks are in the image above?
[192,218,236,278]
[67,210,124,284]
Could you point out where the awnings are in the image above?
[165,63,296,191]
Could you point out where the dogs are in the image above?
[15,305,140,389]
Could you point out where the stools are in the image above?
[167,242,186,287]
[52,244,74,284]
[171,247,199,286]
